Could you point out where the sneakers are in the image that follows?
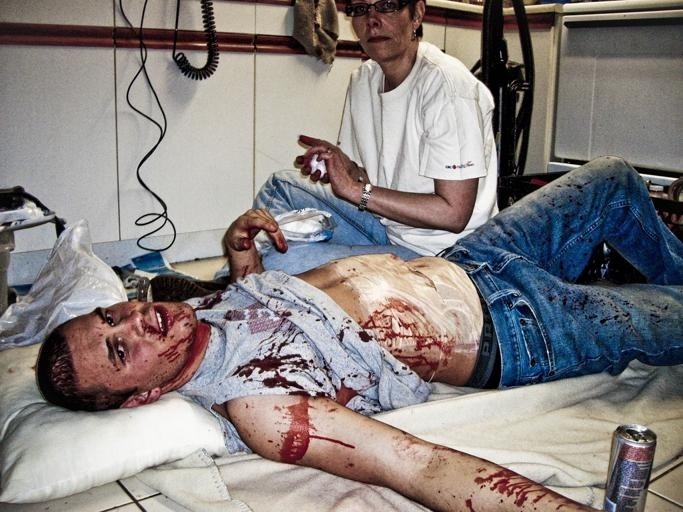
[150,275,213,303]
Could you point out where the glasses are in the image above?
[345,0,410,17]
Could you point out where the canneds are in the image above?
[603,421,657,512]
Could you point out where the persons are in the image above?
[217,0,500,276]
[38,154,683,512]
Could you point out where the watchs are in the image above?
[359,183,371,210]
